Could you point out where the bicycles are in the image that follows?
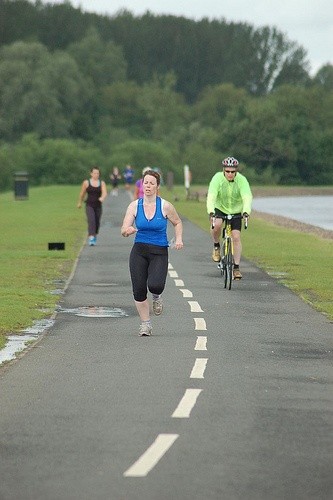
[209,212,249,291]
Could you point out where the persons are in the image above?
[111,163,121,191]
[122,164,136,190]
[136,166,153,198]
[207,157,253,280]
[78,166,107,245]
[121,170,183,336]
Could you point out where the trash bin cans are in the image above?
[14,171,29,199]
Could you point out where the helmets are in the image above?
[222,157,239,167]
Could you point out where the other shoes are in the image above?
[94,237,96,243]
[89,238,94,246]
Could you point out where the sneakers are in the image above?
[152,295,163,315]
[212,246,220,261]
[138,322,152,335]
[233,269,242,277]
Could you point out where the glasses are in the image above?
[225,170,236,173]
